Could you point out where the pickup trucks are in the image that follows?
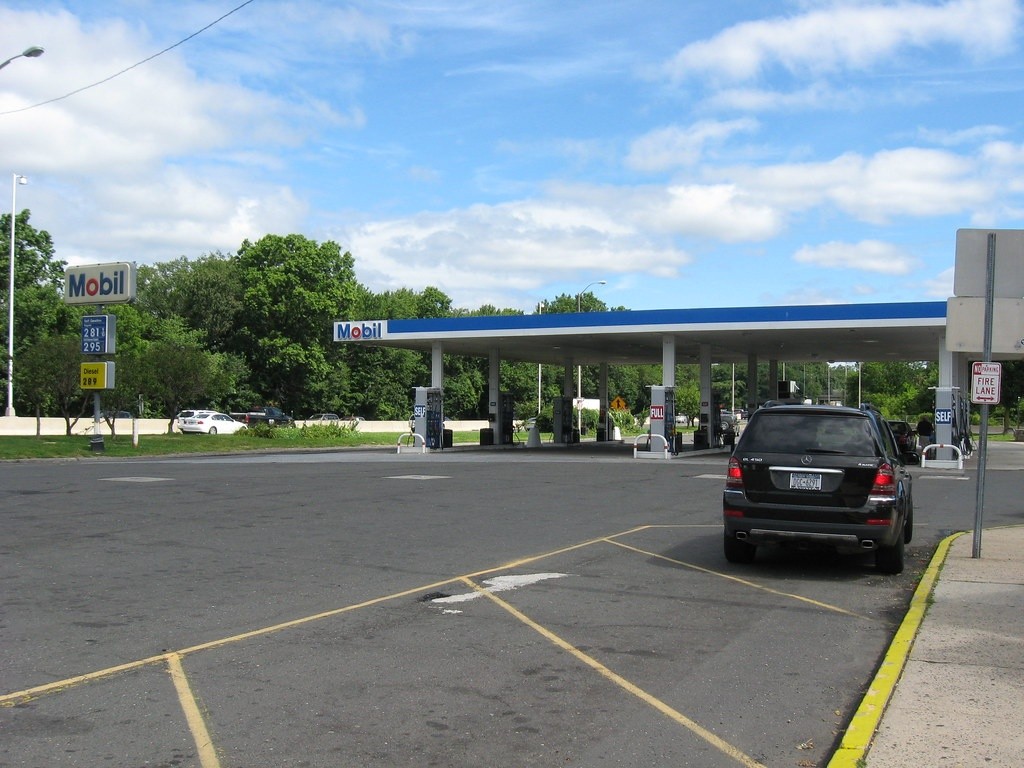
[228,406,293,427]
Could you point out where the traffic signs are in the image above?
[969,362,1001,405]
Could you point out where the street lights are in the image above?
[5,173,29,416]
[857,362,865,409]
[537,301,545,413]
[578,280,608,442]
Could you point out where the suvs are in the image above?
[307,413,339,420]
[722,399,920,575]
[177,409,227,434]
[408,413,446,433]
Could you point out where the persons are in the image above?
[917,415,934,454]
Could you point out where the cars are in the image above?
[341,416,365,421]
[720,408,749,446]
[183,412,248,435]
[887,419,918,452]
[524,417,554,433]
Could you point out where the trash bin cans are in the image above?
[693,430,708,449]
[675,431,683,452]
[443,429,453,447]
[480,429,493,445]
[573,428,580,443]
[596,428,607,442]
